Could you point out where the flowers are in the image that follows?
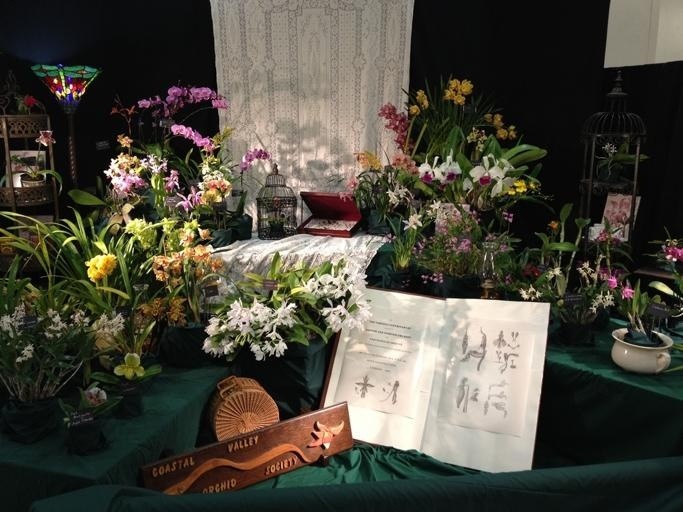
[108,85,235,215]
[3,300,126,363]
[200,264,356,363]
[335,72,529,281]
[602,144,619,158]
[110,348,144,381]
[84,207,211,315]
[235,146,272,175]
[81,385,111,412]
[518,217,563,301]
[660,236,682,265]
[579,257,636,313]
[592,220,624,249]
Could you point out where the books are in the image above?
[316,283,551,475]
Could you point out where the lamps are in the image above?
[29,62,105,193]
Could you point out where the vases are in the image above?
[648,296,681,328]
[6,393,60,444]
[478,241,501,297]
[609,327,673,374]
